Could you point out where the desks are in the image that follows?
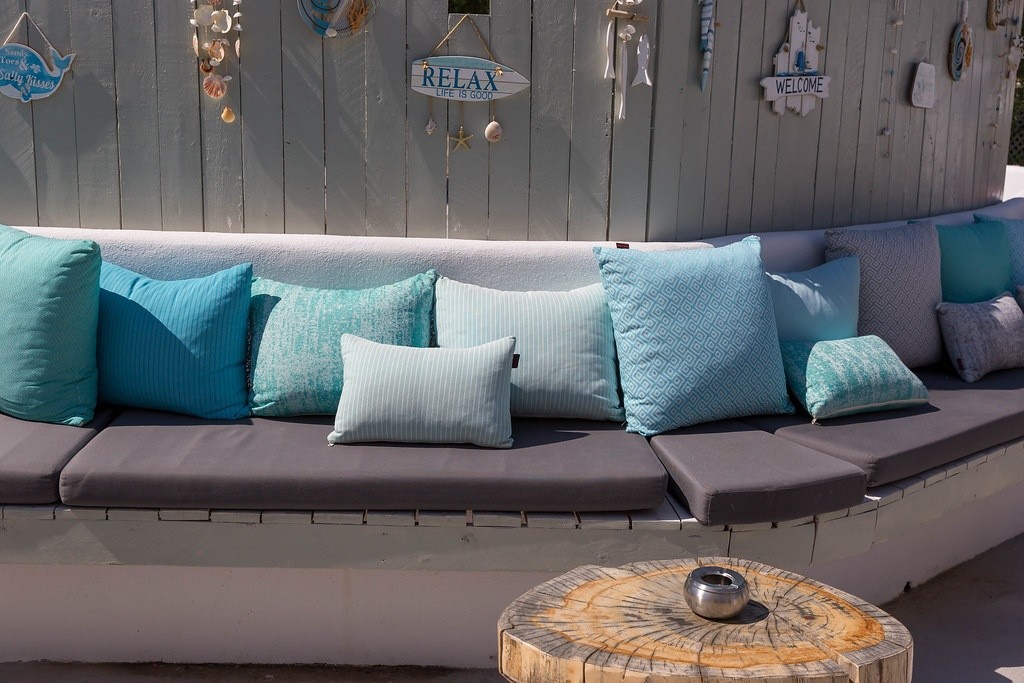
[497,558,913,683]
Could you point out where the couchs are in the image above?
[0,197,1024,667]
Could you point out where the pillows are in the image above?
[433,273,624,422]
[248,269,435,416]
[99,261,253,419]
[780,335,930,424]
[936,291,1024,383]
[908,220,1012,304]
[0,224,101,425]
[327,332,515,447]
[592,235,795,436]
[765,255,860,341]
[973,212,1024,292]
[825,221,942,369]
